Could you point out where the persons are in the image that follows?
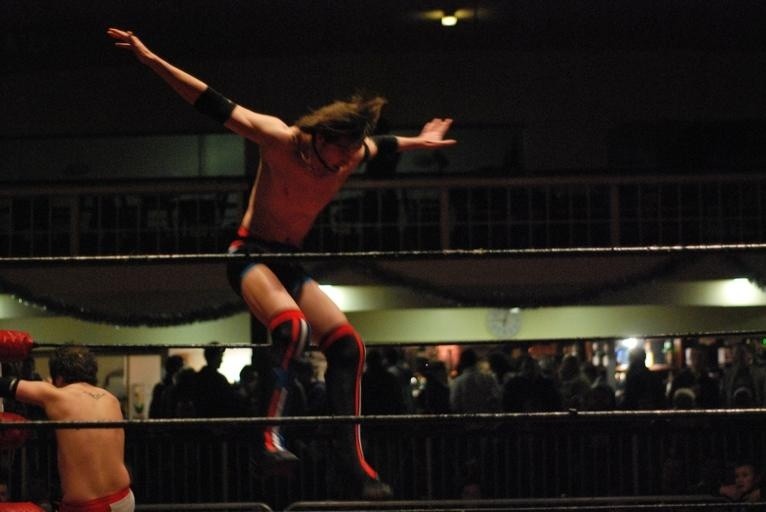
[283,339,766,512]
[1,338,135,512]
[105,25,457,485]
[147,343,274,503]
